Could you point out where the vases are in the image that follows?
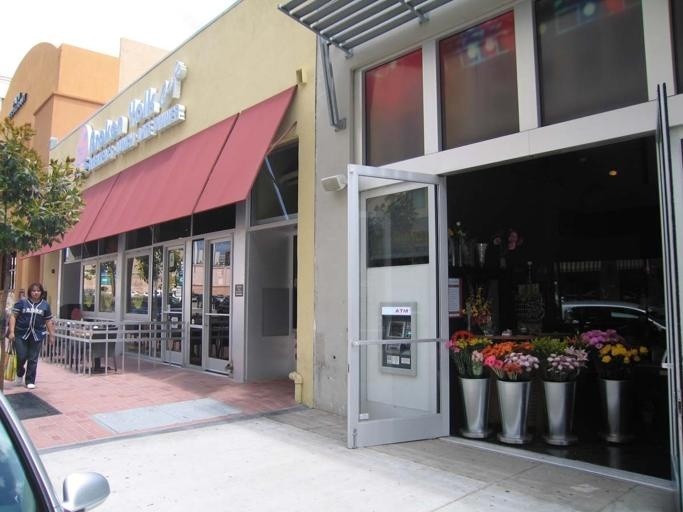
[456,373,638,446]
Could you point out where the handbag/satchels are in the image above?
[4,352,17,381]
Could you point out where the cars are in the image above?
[0,389,111,512]
[562,299,666,368]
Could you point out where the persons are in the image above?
[8,282,56,389]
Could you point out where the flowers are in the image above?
[446,221,523,253]
[442,288,651,383]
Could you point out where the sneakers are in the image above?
[16,376,23,384]
[26,383,36,389]
